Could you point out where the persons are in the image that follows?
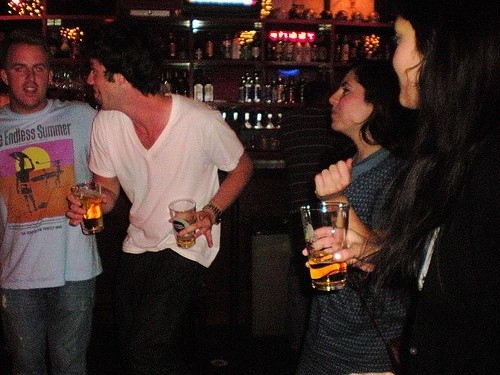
[295,61,414,375]
[302,0,500,375]
[62,16,251,375]
[280,81,357,254]
[1,34,101,375]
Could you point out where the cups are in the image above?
[299,201,350,292]
[168,199,197,250]
[74,182,105,234]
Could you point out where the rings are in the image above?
[332,226,336,238]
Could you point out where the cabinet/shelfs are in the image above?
[0,1,397,151]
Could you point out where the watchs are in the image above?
[203,204,223,226]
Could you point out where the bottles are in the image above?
[193,32,241,59]
[335,33,361,62]
[49,66,84,91]
[251,36,261,61]
[158,31,187,59]
[237,71,310,105]
[194,72,215,103]
[49,30,89,60]
[161,70,187,97]
[296,40,311,62]
[219,109,282,153]
